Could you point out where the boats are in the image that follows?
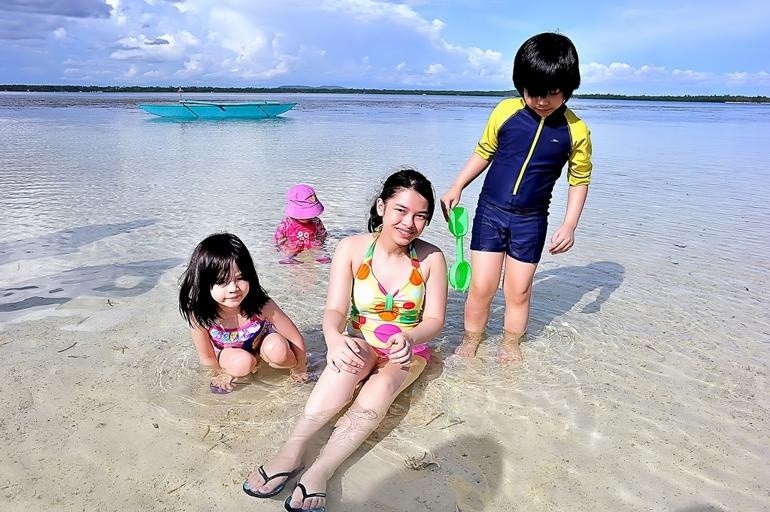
[137,100,297,119]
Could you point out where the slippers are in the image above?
[242,458,304,498]
[284,475,327,512]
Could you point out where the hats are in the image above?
[284,184,324,220]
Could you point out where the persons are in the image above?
[241,168,450,511]
[272,181,333,264]
[439,31,593,366]
[176,232,319,396]
